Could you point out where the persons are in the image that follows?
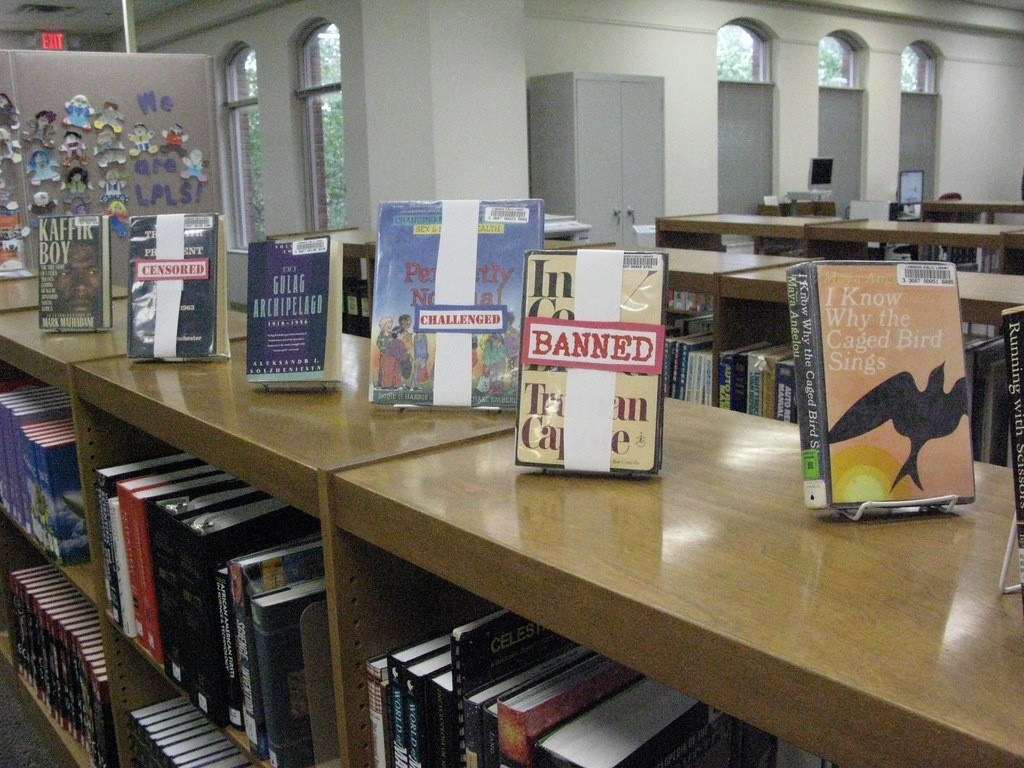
[46,228,102,315]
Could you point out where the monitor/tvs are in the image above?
[812,158,833,184]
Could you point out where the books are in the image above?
[38,215,113,331]
[2,564,112,768]
[127,212,231,358]
[662,289,798,425]
[1003,305,1024,606]
[365,606,837,768]
[514,250,670,473]
[786,261,977,510]
[963,335,1013,468]
[0,386,88,565]
[368,198,544,408]
[246,235,343,382]
[93,453,327,768]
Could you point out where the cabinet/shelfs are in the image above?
[0,201,1024,768]
[529,70,667,248]
